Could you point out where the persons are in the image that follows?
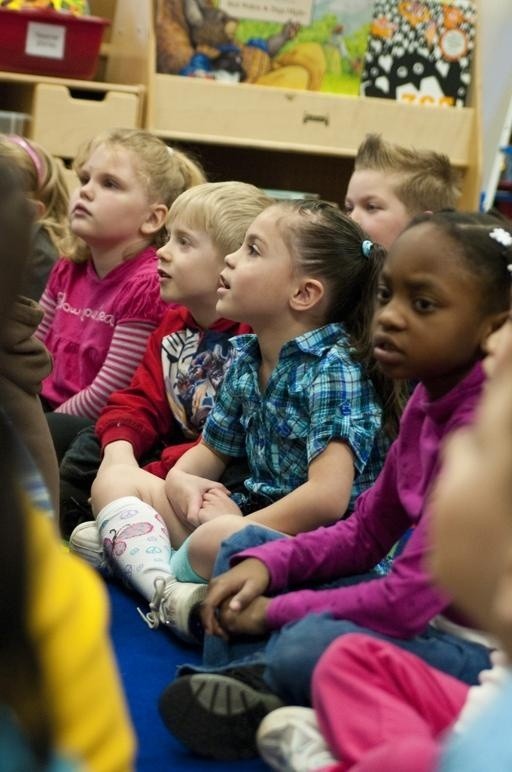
[1,130,512,772]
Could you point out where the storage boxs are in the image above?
[0,4,113,80]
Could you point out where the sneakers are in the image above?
[68,521,122,579]
[150,582,208,645]
[157,673,337,772]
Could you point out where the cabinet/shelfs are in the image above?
[151,73,482,215]
[0,70,149,163]
